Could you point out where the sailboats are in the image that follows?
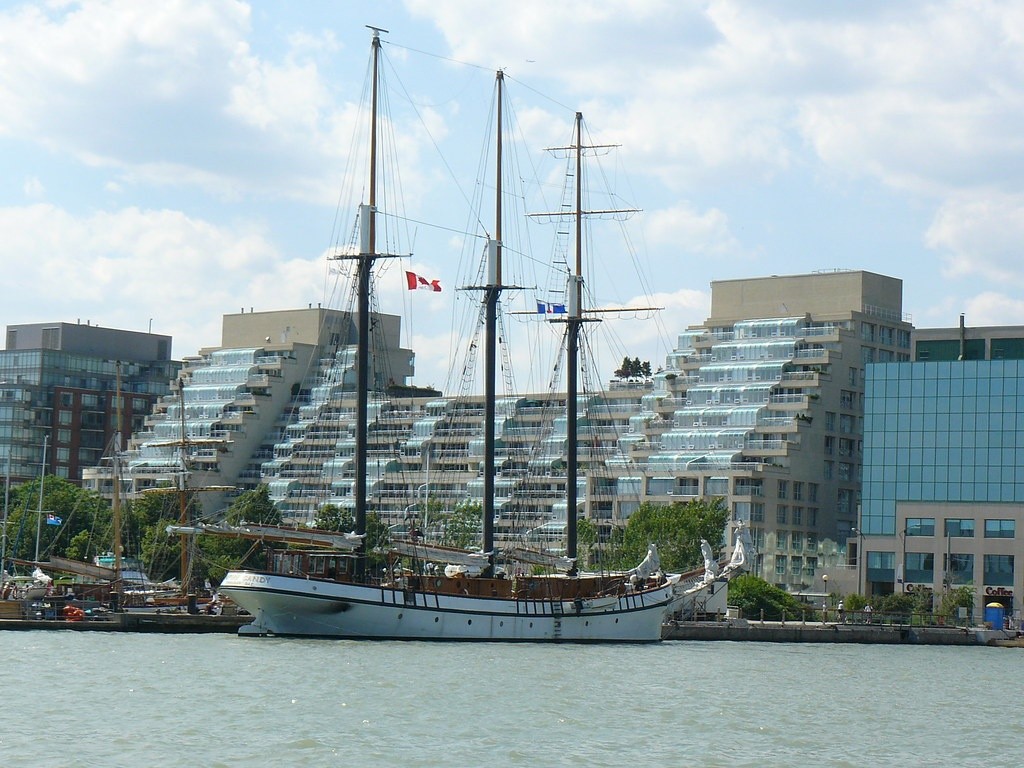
[164,22,677,644]
[1,359,255,634]
[379,66,752,616]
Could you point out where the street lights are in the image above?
[851,527,862,596]
[822,574,829,622]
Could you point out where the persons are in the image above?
[574,592,585,616]
[823,601,827,624]
[205,588,219,614]
[204,578,211,589]
[863,602,873,624]
[837,600,845,623]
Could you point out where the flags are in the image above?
[405,270,443,292]
[536,299,568,315]
[47,514,63,525]
